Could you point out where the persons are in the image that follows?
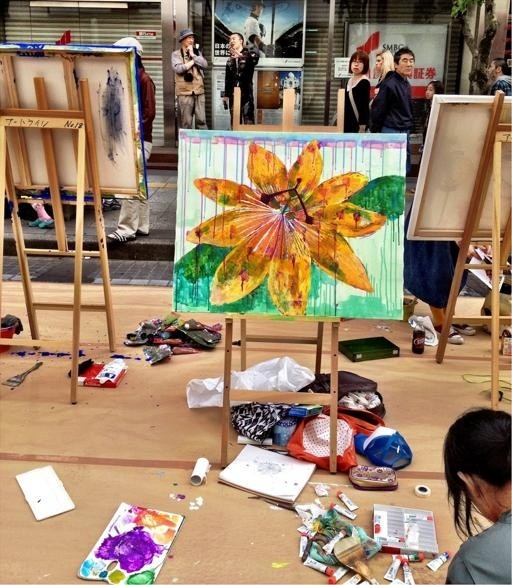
[105,36,157,242]
[487,58,512,95]
[341,51,373,133]
[27,200,57,228]
[442,407,511,584]
[422,78,443,147]
[224,33,260,131]
[171,28,210,129]
[404,202,487,344]
[368,48,416,177]
[246,0,277,59]
[367,48,395,108]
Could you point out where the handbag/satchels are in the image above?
[231,371,398,491]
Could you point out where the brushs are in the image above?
[334,536,380,585]
[1,362,43,387]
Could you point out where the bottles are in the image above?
[410,318,427,354]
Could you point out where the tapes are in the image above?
[415,485,431,497]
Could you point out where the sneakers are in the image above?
[107,230,149,242]
[104,200,120,211]
[435,324,476,344]
[29,219,54,229]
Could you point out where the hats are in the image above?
[113,37,143,57]
[178,30,196,42]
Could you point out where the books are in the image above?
[217,444,317,505]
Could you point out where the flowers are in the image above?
[185,140,404,318]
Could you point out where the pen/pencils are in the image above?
[266,499,296,511]
